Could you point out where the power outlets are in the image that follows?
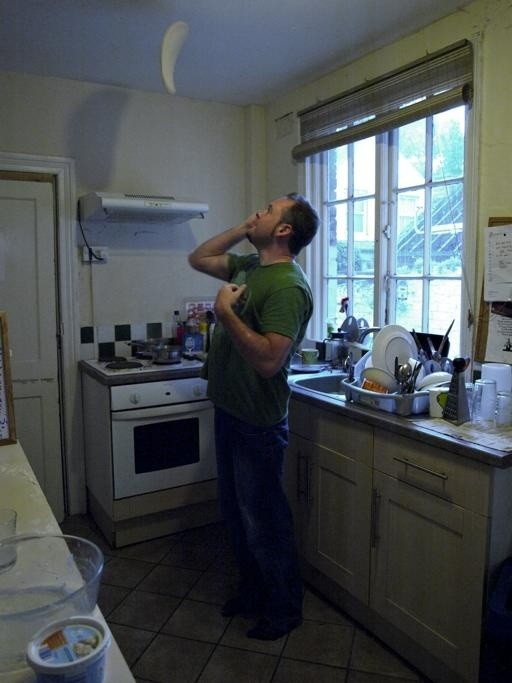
[83,247,107,262]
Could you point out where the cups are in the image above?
[465,379,512,432]
[301,348,319,368]
[429,387,450,418]
[0,507,17,574]
[25,616,112,682]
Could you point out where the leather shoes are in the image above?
[219,590,304,641]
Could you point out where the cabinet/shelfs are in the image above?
[367,425,511,682]
[279,398,376,634]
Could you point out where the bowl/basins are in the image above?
[0,534,105,679]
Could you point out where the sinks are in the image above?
[285,369,349,402]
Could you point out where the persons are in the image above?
[188,192,319,640]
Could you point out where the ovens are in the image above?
[111,374,218,523]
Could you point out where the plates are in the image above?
[290,364,322,372]
[360,325,453,392]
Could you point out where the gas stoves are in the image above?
[80,355,203,386]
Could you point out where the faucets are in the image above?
[357,326,382,345]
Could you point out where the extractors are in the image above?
[77,194,209,225]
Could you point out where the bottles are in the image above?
[172,303,215,352]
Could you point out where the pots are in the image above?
[128,339,183,364]
